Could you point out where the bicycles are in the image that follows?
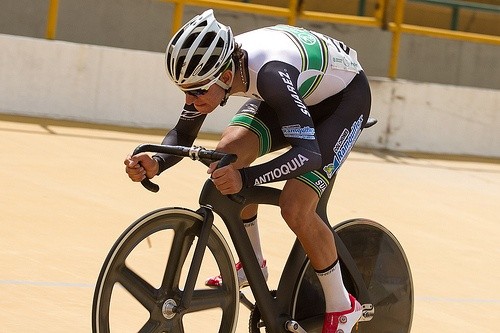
[89,143,415,333]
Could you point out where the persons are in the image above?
[124,10,372,333]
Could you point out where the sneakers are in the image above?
[322,292,362,333]
[205,258,268,287]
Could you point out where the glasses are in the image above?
[181,58,232,96]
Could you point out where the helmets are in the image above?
[164,9,235,84]
